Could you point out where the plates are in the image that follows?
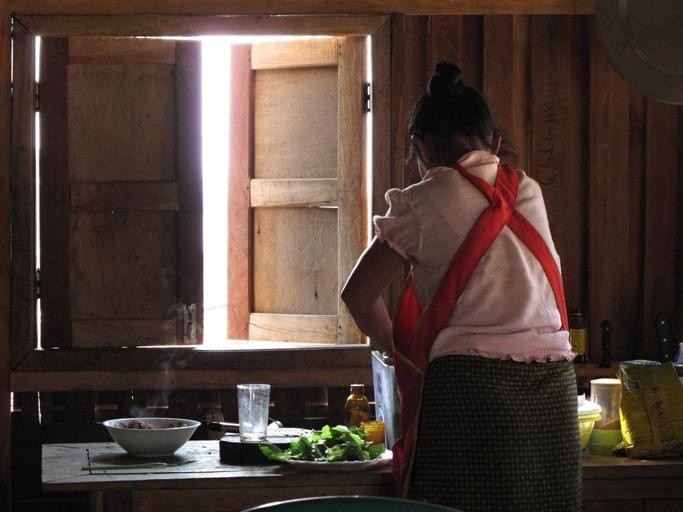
[276,444,392,472]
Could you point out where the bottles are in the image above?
[567,298,591,362]
[343,384,370,428]
[358,420,384,446]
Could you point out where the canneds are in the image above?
[569,312,588,363]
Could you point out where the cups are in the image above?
[236,383,270,443]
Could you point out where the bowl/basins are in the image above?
[102,415,201,458]
[578,402,602,451]
[588,428,621,456]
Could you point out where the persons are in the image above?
[338,62,583,509]
